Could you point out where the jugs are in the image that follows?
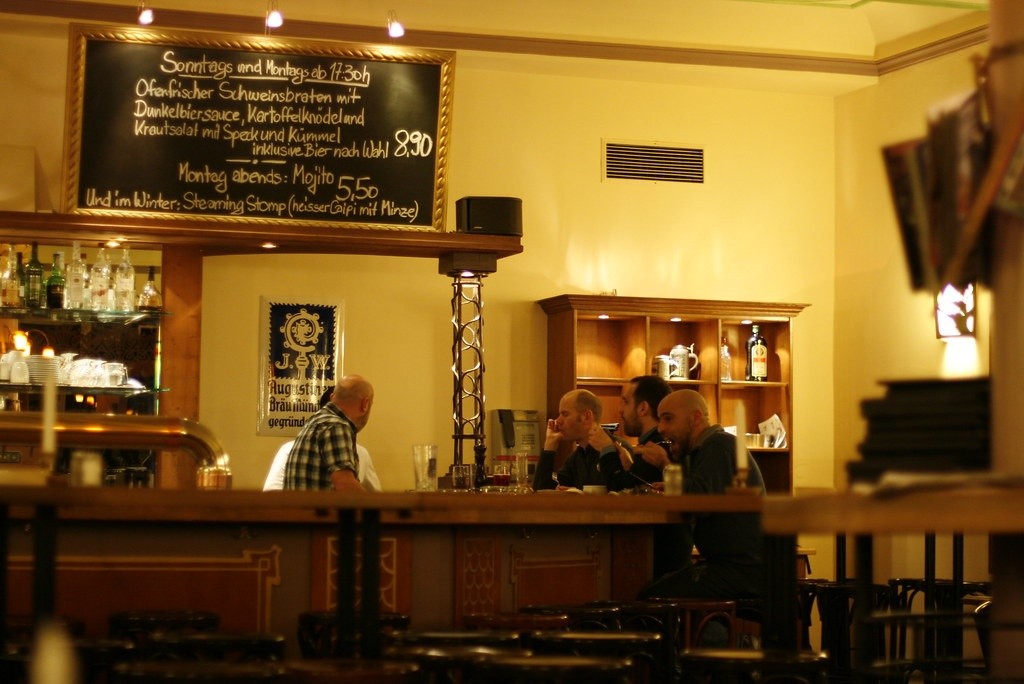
[654,343,698,381]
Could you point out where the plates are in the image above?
[25,355,63,387]
[583,485,607,494]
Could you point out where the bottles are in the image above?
[68,451,150,489]
[0,239,138,312]
[721,338,732,380]
[746,325,768,382]
[140,265,163,312]
[195,459,232,488]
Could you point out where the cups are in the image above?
[492,464,511,486]
[515,451,529,487]
[453,465,470,492]
[745,433,765,447]
[414,444,438,492]
[663,464,683,495]
[0,350,29,383]
[62,353,129,386]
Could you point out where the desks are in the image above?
[763,485,1023,536]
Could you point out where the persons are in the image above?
[283,373,374,492]
[532,375,800,622]
[263,383,382,491]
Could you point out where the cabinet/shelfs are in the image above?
[1,230,166,484]
[534,293,817,650]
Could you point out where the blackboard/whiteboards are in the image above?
[64,20,456,239]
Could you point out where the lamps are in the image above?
[935,284,980,339]
[8,327,55,358]
[137,0,154,26]
[387,8,404,44]
[264,0,284,35]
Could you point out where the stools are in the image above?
[1,577,994,684]
